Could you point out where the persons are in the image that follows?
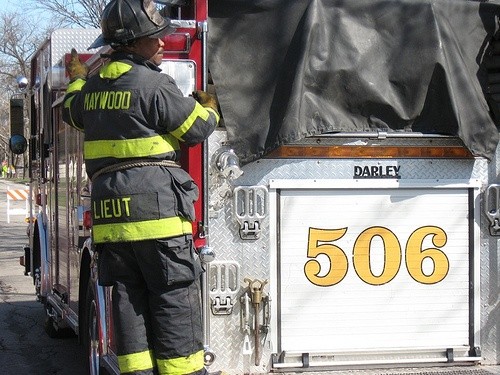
[59,0,222,375]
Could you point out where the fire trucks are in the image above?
[9,1,500,375]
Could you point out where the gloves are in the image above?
[66,48,90,81]
[188,91,221,116]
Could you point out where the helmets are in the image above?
[87,0,177,50]
